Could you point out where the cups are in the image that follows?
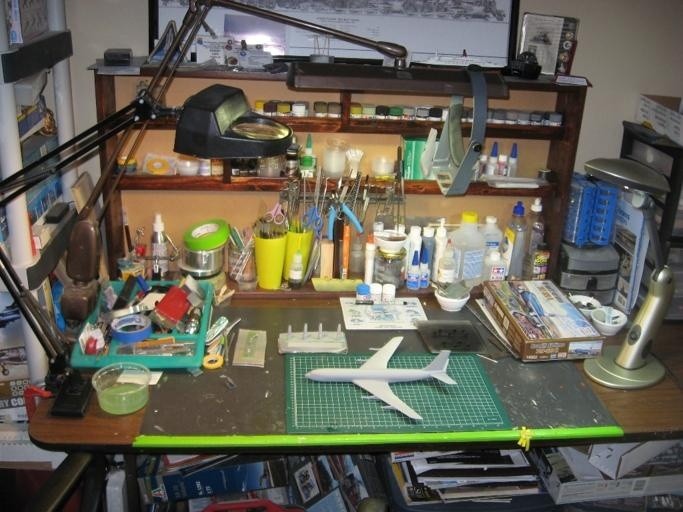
[371,160,394,175]
[320,150,348,178]
[222,225,314,292]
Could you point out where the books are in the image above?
[389,452,540,505]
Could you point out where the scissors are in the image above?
[302,165,323,230]
[263,197,285,225]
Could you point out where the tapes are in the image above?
[203,355,223,369]
[110,313,153,344]
[183,218,230,250]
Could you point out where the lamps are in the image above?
[0,84,294,419]
[59,0,408,326]
[581,156,678,390]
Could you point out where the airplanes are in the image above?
[299,332,463,423]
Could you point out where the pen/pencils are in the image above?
[228,224,254,281]
[207,329,235,362]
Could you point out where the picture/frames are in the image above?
[147,1,520,69]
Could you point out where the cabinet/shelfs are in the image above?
[0,0,79,472]
[620,120,683,321]
[27,54,683,510]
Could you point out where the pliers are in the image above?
[328,193,364,240]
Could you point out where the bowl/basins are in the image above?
[434,289,471,314]
[567,292,627,338]
[90,362,151,416]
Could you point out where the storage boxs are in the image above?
[619,93,683,237]
[4,0,48,45]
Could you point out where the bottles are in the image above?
[348,198,551,304]
[300,134,317,178]
[149,211,170,278]
[248,100,563,132]
[479,142,522,179]
